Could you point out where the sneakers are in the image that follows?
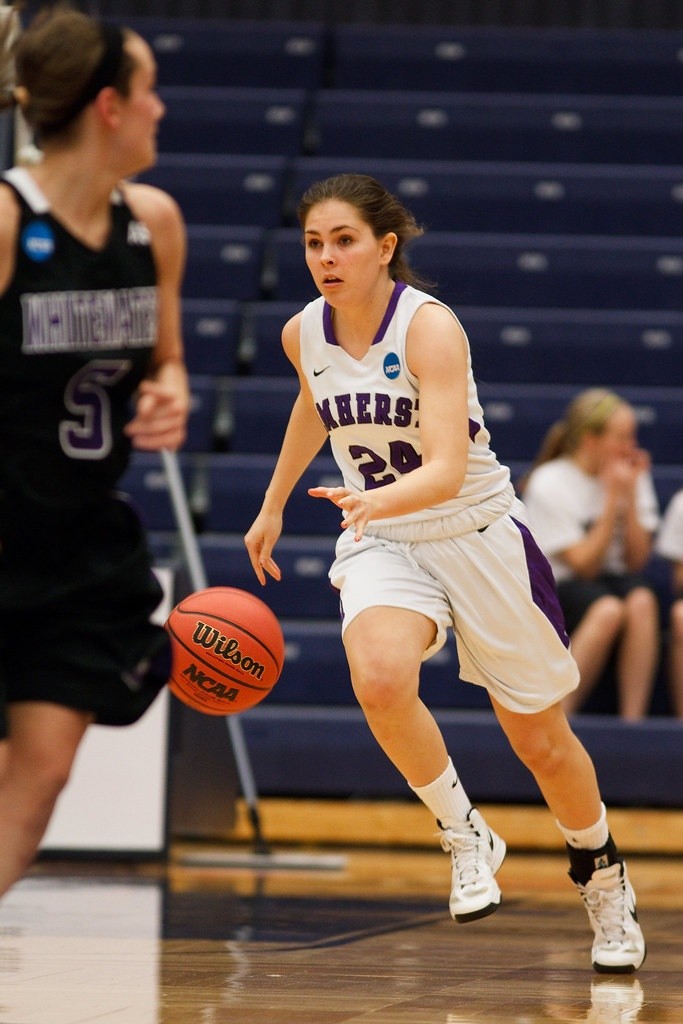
[437,808,507,923]
[567,861,646,972]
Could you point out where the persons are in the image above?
[515,390,660,718]
[243,176,645,974]
[653,488,683,718]
[1,15,188,906]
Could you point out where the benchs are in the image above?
[0,0,683,803]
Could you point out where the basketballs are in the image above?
[160,584,286,719]
